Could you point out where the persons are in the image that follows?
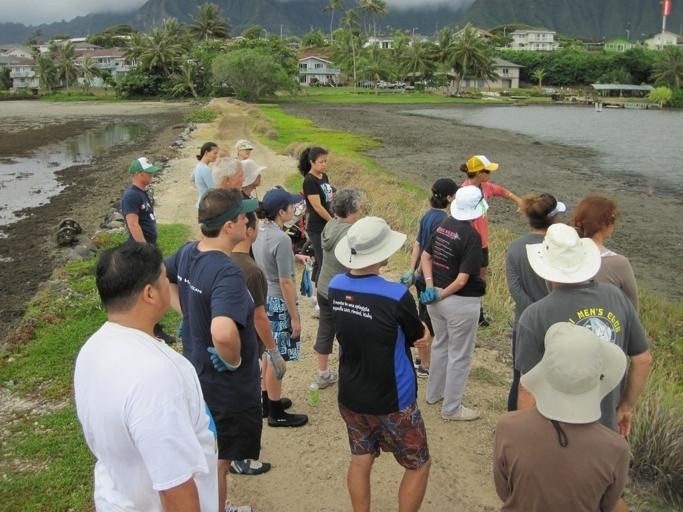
[492,321,632,512]
[515,223,653,512]
[420,185,489,421]
[72,241,220,511]
[241,158,267,200]
[566,195,640,315]
[327,216,435,512]
[460,154,524,327]
[163,187,263,512]
[196,156,246,211]
[235,139,254,161]
[121,156,176,345]
[230,211,287,476]
[401,178,463,377]
[189,140,219,209]
[313,188,363,389]
[298,146,334,318]
[504,191,566,412]
[251,188,313,428]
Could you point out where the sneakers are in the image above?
[268,409,308,427]
[229,459,271,475]
[263,398,292,416]
[153,324,176,343]
[312,306,321,319]
[442,405,481,420]
[318,368,337,389]
[414,356,421,369]
[225,500,252,512]
[417,366,430,377]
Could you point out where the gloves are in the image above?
[208,346,242,372]
[264,346,286,380]
[420,288,434,305]
[307,263,312,297]
[413,269,421,284]
[300,264,307,296]
[400,270,413,287]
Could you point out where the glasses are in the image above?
[475,185,484,209]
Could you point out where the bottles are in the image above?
[308,384,320,408]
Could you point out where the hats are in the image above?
[450,186,489,221]
[129,157,160,173]
[431,178,463,197]
[525,223,601,283]
[546,196,566,218]
[466,155,499,172]
[204,195,259,227]
[262,185,303,217]
[520,321,627,425]
[240,159,267,187]
[236,139,254,152]
[334,216,407,269]
